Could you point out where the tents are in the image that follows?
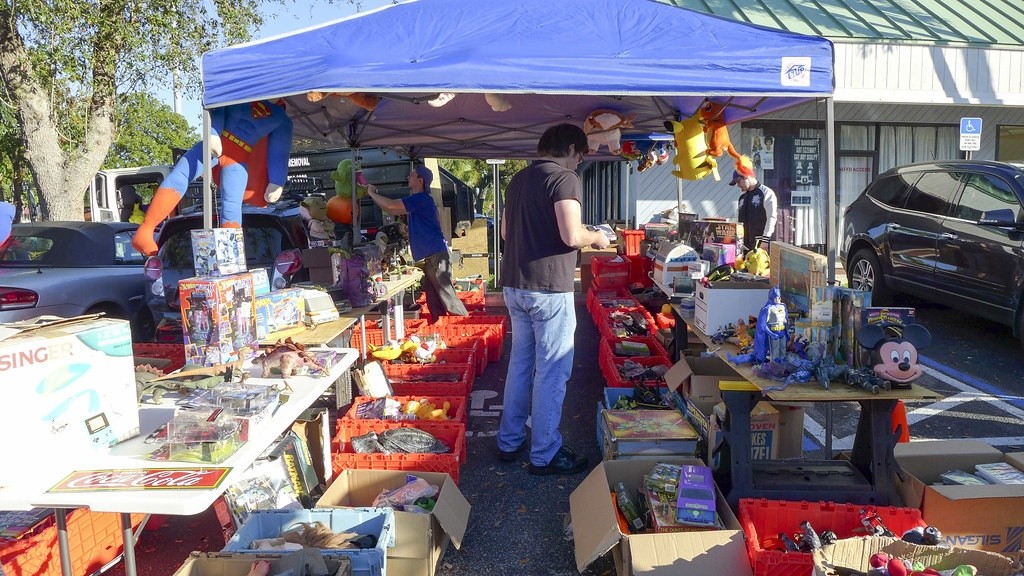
[200,0,838,321]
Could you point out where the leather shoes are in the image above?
[496,440,532,462]
[529,446,588,475]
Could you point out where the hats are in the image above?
[415,165,433,194]
[729,170,744,186]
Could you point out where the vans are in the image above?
[89,165,204,224]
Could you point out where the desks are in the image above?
[255,270,425,361]
[26,347,359,576]
[649,277,938,461]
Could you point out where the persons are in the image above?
[495,123,610,475]
[367,164,468,325]
[754,287,791,364]
[752,136,773,170]
[727,351,754,366]
[729,169,778,274]
[139,198,163,232]
[848,368,891,395]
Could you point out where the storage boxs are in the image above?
[0,217,1024,576]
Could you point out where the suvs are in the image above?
[836,160,1024,344]
[143,197,329,326]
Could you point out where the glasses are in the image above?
[735,176,742,184]
[568,143,585,163]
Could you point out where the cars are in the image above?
[0,223,160,335]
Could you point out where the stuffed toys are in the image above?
[306,90,380,112]
[375,220,409,270]
[734,247,770,277]
[429,93,512,112]
[399,395,450,421]
[367,335,446,364]
[584,102,752,180]
[300,159,367,247]
[338,249,377,309]
[131,97,293,256]
